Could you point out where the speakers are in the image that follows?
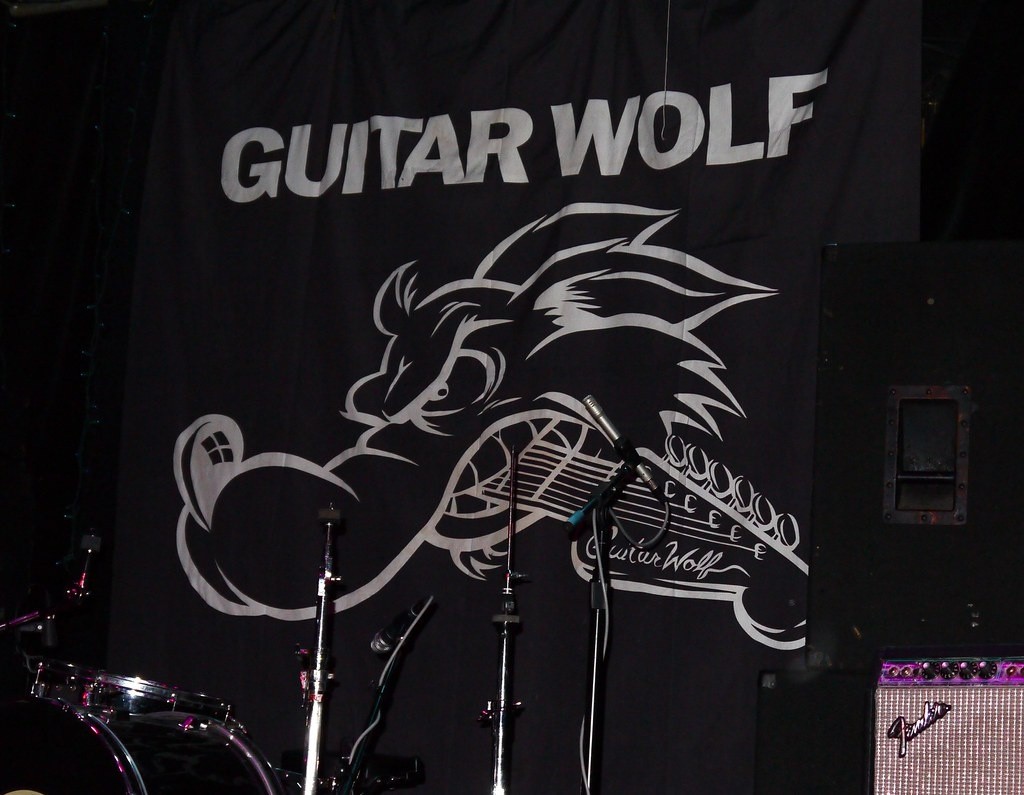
[753,242,1024,795]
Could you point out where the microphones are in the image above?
[583,394,669,503]
[75,527,101,605]
[370,598,428,654]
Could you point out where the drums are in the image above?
[0,657,304,795]
